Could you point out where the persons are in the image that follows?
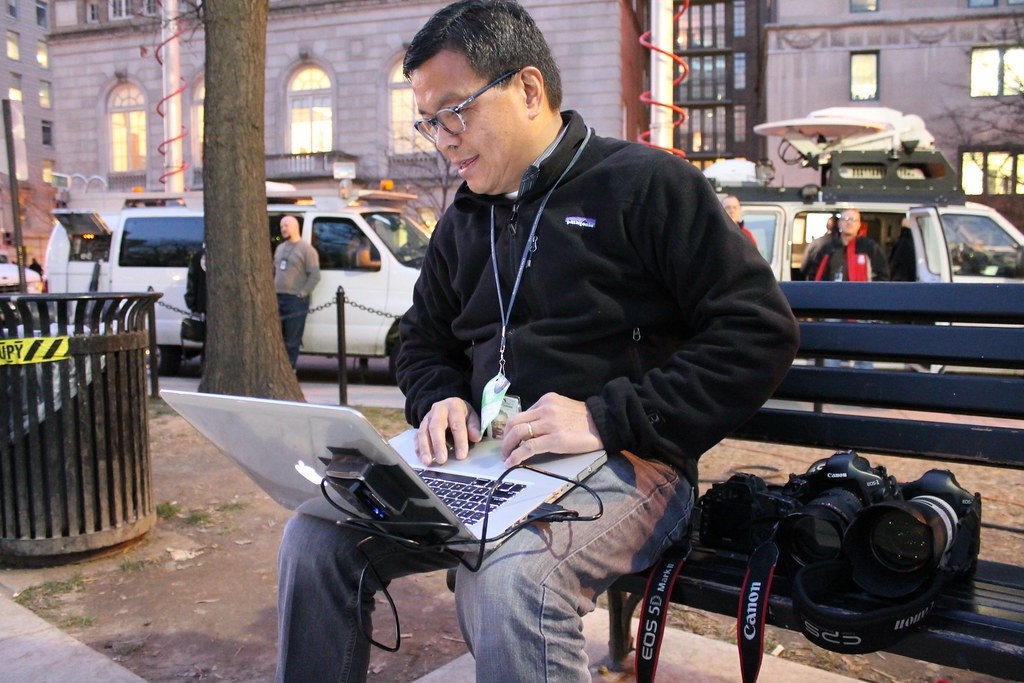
[490,409,508,439]
[799,206,892,370]
[273,215,321,368]
[29,258,42,276]
[272,0,800,683]
[182,250,208,315]
[720,192,758,248]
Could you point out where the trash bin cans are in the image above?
[0,291,164,567]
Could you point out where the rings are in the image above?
[527,422,534,438]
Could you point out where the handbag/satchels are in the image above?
[180,311,208,341]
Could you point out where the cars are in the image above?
[0,257,43,296]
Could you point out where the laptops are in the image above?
[158,389,608,557]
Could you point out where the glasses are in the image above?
[839,216,858,222]
[414,69,519,143]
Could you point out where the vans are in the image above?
[40,207,122,326]
[106,188,435,382]
[708,140,1024,375]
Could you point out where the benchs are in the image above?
[448,283,1024,683]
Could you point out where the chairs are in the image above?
[316,243,349,270]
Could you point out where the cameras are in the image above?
[698,449,982,654]
